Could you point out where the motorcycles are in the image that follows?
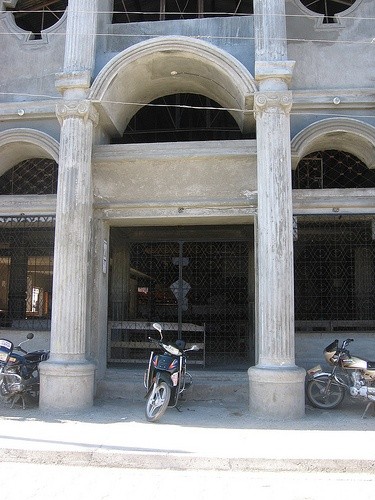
[305,338,375,419]
[0,333,50,410]
[144,323,200,422]
